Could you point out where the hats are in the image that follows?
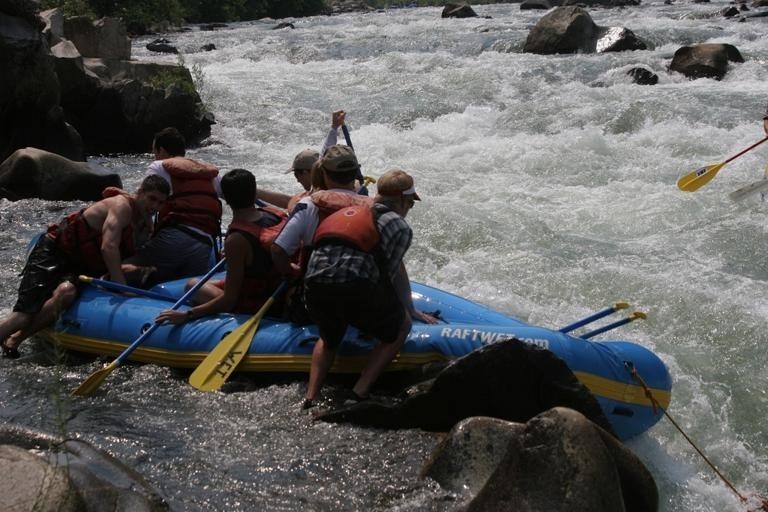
[283,149,319,175]
[312,144,359,187]
[377,169,421,202]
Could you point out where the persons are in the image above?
[2,175,171,358]
[120,110,369,325]
[302,171,438,409]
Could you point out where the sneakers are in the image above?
[1,338,21,359]
[301,395,323,413]
[343,390,377,406]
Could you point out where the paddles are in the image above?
[189,176,376,391]
[71,257,226,398]
[678,134,766,192]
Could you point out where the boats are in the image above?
[20,224,673,444]
